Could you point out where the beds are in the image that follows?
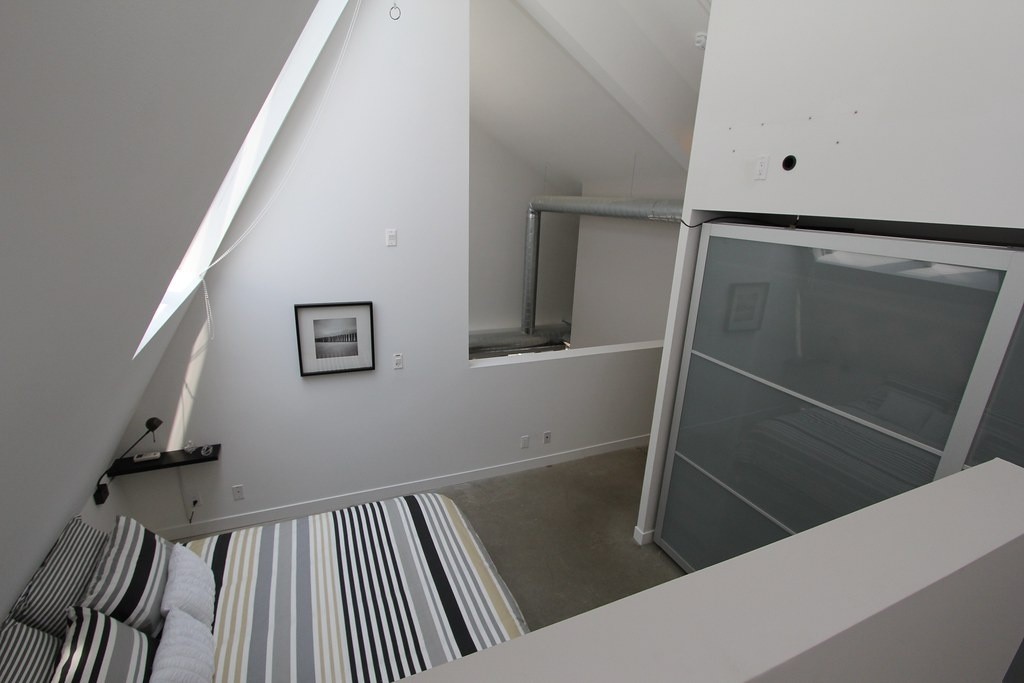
[0,493,533,683]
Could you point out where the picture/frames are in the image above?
[294,301,376,377]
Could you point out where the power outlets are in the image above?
[521,435,529,449]
[544,431,551,444]
[232,484,245,501]
[190,493,202,507]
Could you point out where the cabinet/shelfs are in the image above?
[652,222,1023,576]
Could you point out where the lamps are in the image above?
[94,417,164,505]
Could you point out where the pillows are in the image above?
[0,615,62,683]
[9,515,109,640]
[161,542,216,629]
[75,515,176,642]
[49,607,161,683]
[148,607,217,683]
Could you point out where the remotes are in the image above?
[134,452,160,462]
